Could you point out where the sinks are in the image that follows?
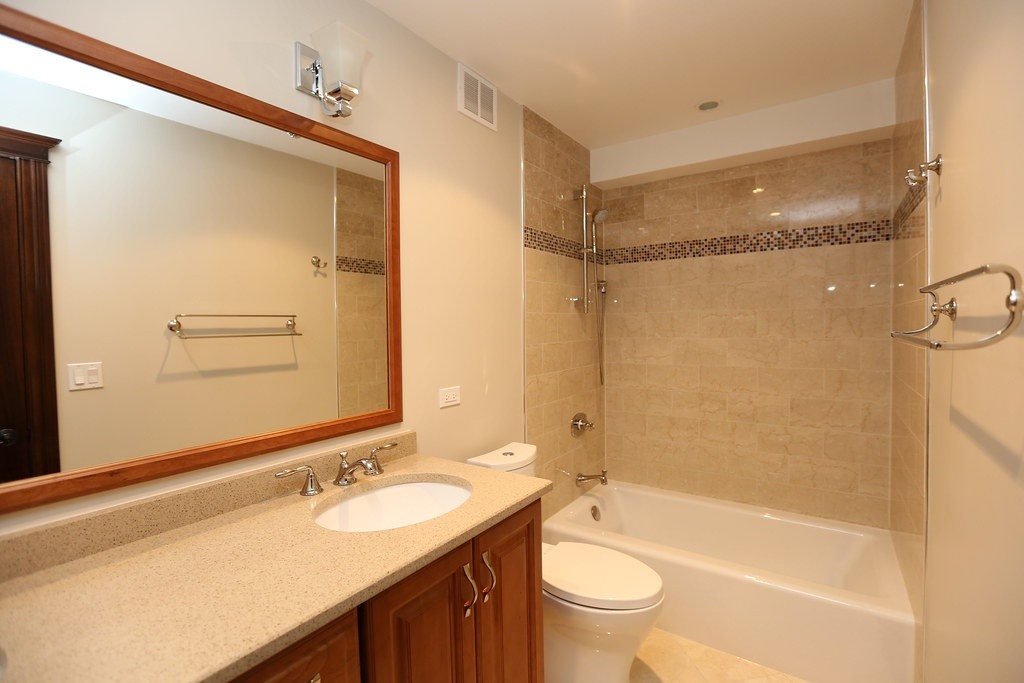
[311,472,473,533]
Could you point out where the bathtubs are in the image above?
[542,477,914,682]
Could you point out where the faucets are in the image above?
[333,451,377,485]
[574,468,609,487]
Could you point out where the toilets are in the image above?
[466,441,667,683]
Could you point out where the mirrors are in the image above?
[0,4,404,513]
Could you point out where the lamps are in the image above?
[294,40,359,120]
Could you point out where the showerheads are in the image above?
[590,207,610,254]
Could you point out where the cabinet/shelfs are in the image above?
[363,497,546,683]
[226,607,361,683]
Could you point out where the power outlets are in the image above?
[439,386,461,410]
